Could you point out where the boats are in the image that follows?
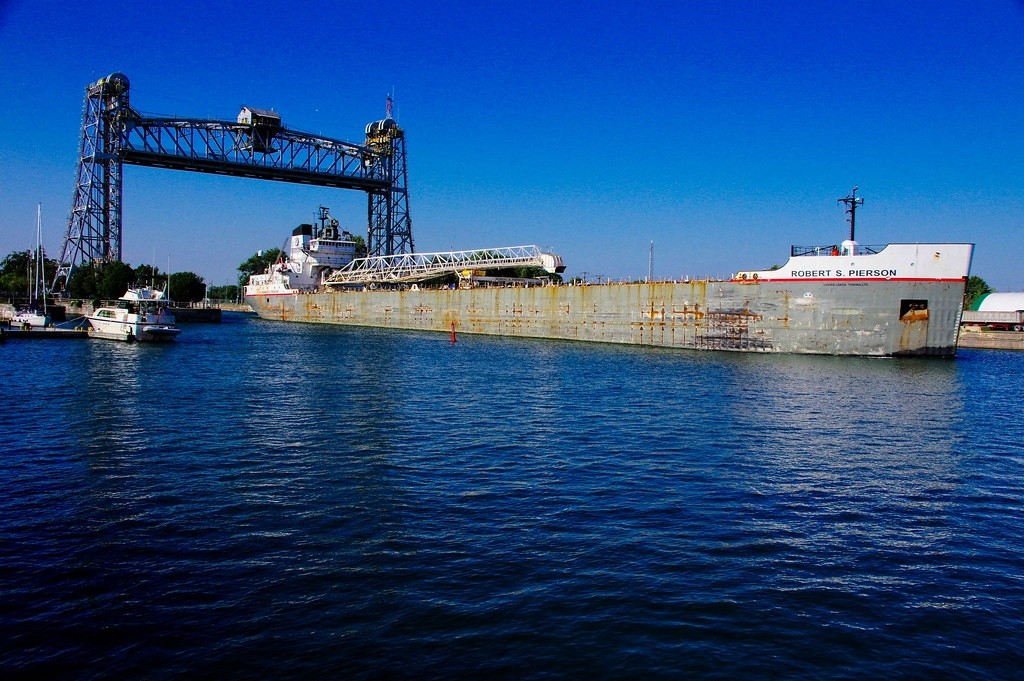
[84,278,181,342]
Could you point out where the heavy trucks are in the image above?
[962,309,1024,331]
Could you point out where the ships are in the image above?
[243,184,976,359]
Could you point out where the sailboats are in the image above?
[10,202,51,326]
